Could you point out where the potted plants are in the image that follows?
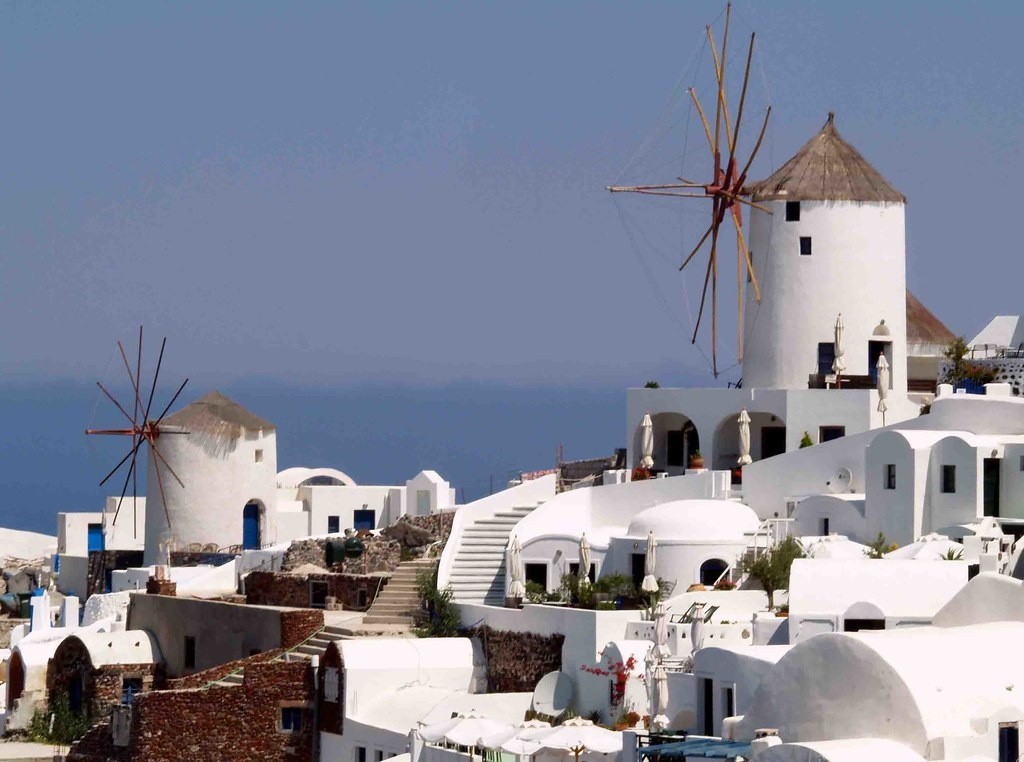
[613,695,650,730]
[690,448,703,467]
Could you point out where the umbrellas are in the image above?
[876,352,889,427]
[641,530,659,605]
[578,532,591,592]
[738,407,752,466]
[691,603,705,656]
[509,535,525,598]
[832,313,846,389]
[651,601,671,664]
[640,412,654,470]
[644,646,652,714]
[653,663,670,732]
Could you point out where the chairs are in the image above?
[670,602,721,624]
